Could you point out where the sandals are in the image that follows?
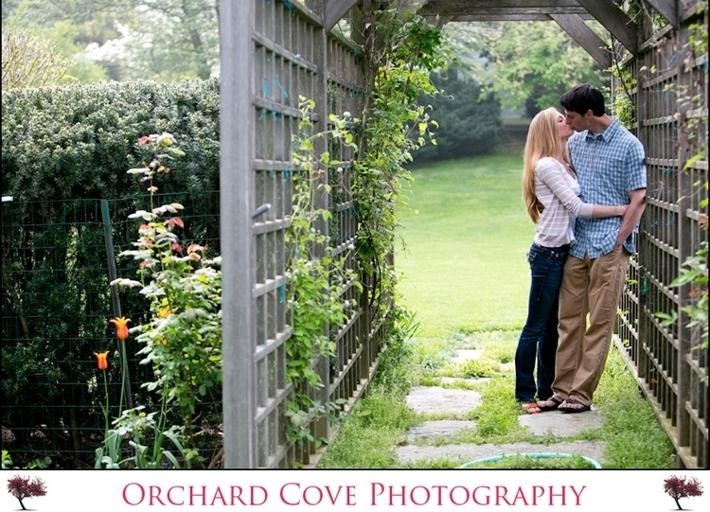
[520,399,542,414]
[538,396,563,411]
[557,398,591,413]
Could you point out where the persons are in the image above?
[515,106,629,416]
[536,83,647,411]
